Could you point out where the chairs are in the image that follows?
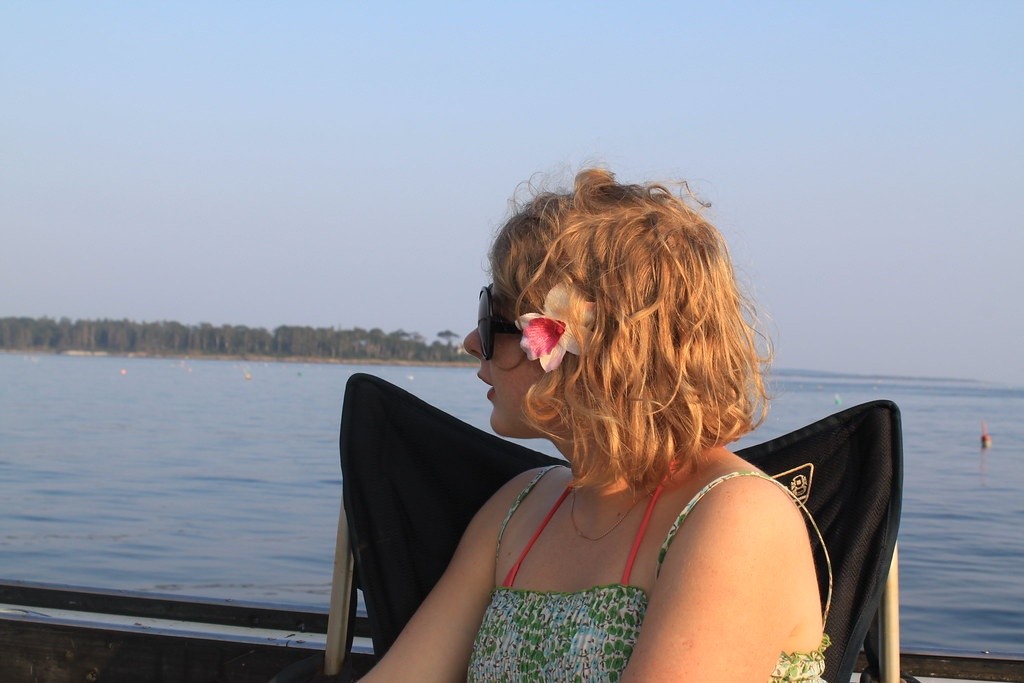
[323,371,905,683]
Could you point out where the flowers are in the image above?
[515,279,599,373]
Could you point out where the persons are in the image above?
[355,171,823,683]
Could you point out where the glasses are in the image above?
[479,284,523,360]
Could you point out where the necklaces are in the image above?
[570,483,636,539]
